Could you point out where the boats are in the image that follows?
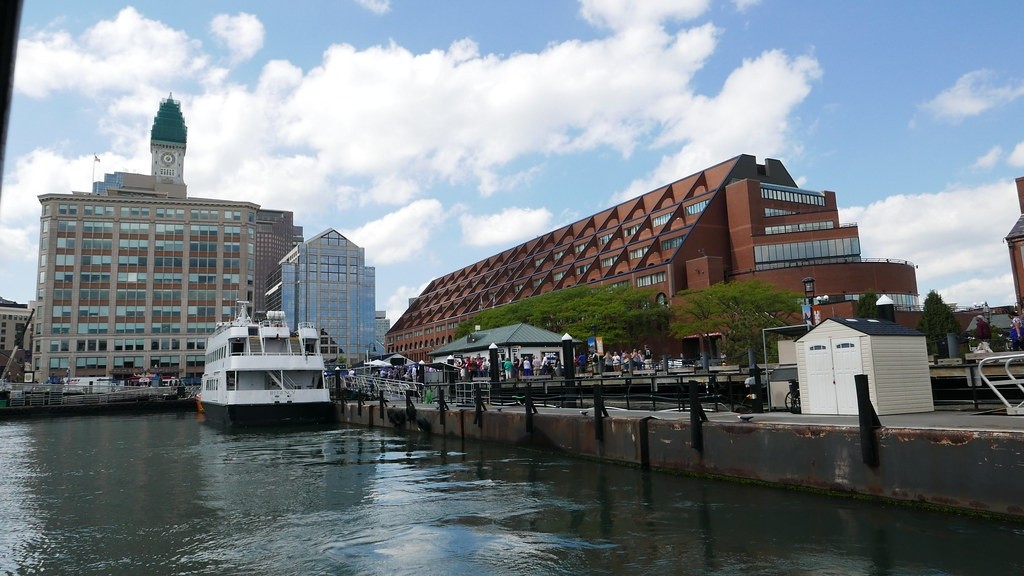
[198,311,332,429]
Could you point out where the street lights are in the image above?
[803,274,816,331]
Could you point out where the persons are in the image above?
[975,315,993,353]
[502,352,548,379]
[1009,311,1024,351]
[577,346,651,373]
[349,369,355,376]
[465,354,492,378]
[376,363,434,382]
